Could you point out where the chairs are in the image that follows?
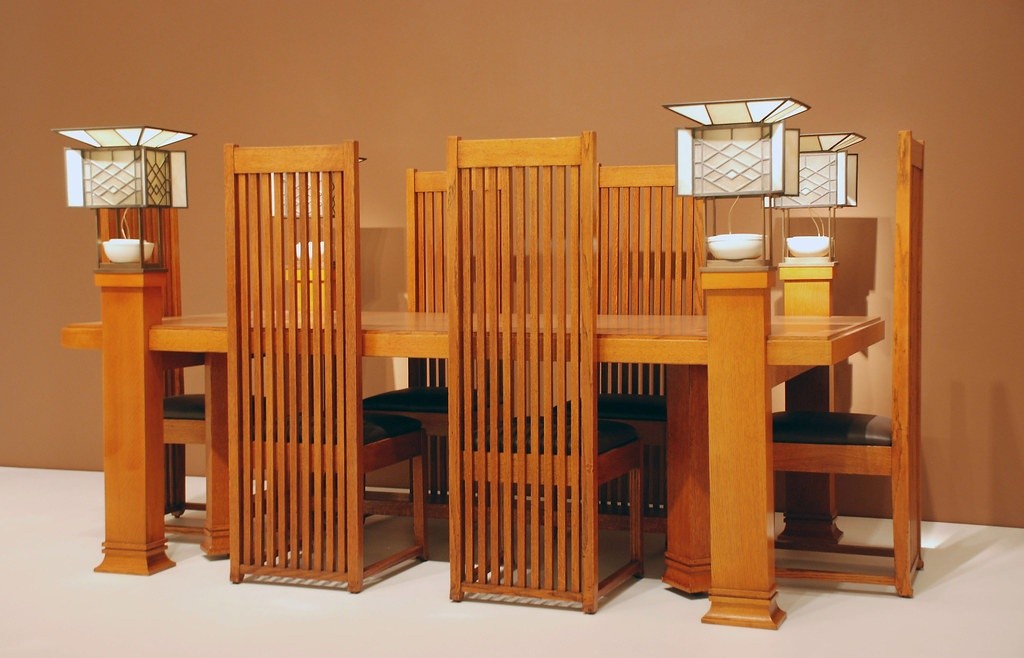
[363,169,449,518]
[101,208,206,535]
[224,139,429,593]
[446,131,645,614]
[774,131,923,597]
[597,163,708,534]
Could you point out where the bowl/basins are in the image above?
[102,239,154,262]
[787,236,833,256]
[708,233,768,261]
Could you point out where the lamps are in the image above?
[763,132,867,267]
[661,95,811,272]
[52,126,197,275]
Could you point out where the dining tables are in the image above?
[62,311,885,595]
